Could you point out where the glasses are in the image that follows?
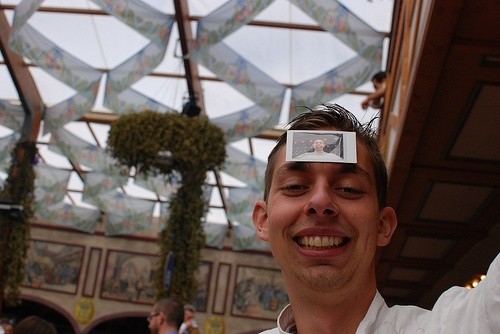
[147,313,160,321]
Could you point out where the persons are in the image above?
[252,102,500,334]
[147,298,186,334]
[178,305,201,334]
[294,138,343,160]
[0,317,55,334]
[361,70,388,109]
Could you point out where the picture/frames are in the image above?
[99,248,215,314]
[230,264,291,322]
[19,238,87,297]
